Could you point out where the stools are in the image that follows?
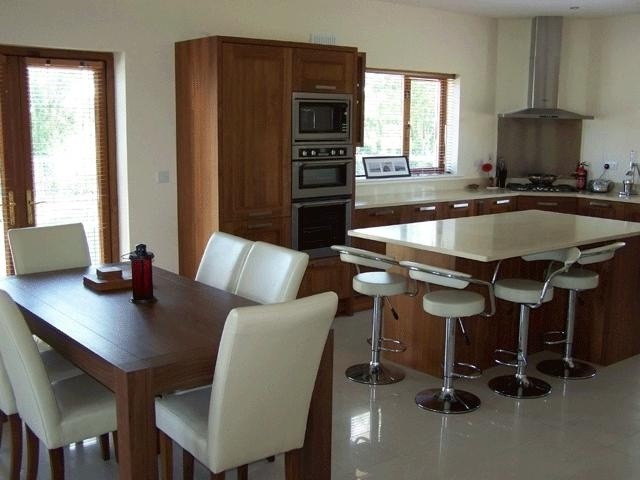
[399,260,496,413]
[487,248,580,400]
[331,244,418,385]
[537,241,624,378]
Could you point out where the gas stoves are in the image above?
[507,182,579,193]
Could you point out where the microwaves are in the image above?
[292,92,350,142]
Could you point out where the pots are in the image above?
[527,173,562,186]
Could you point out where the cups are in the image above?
[624,179,632,197]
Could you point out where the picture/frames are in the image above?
[362,156,411,179]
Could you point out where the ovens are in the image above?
[291,161,354,259]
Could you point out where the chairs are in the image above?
[235,241,310,303]
[8,225,92,274]
[194,232,254,292]
[0,338,81,480]
[1,291,119,478]
[154,290,336,477]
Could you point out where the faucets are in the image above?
[625,150,640,184]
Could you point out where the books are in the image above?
[82,271,133,292]
[97,267,123,278]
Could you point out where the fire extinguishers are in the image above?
[576,161,589,190]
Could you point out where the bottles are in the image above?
[496,155,506,190]
[129,243,155,304]
[576,164,586,189]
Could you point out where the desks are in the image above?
[2,259,261,478]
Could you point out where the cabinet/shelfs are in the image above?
[293,42,358,93]
[174,36,292,281]
